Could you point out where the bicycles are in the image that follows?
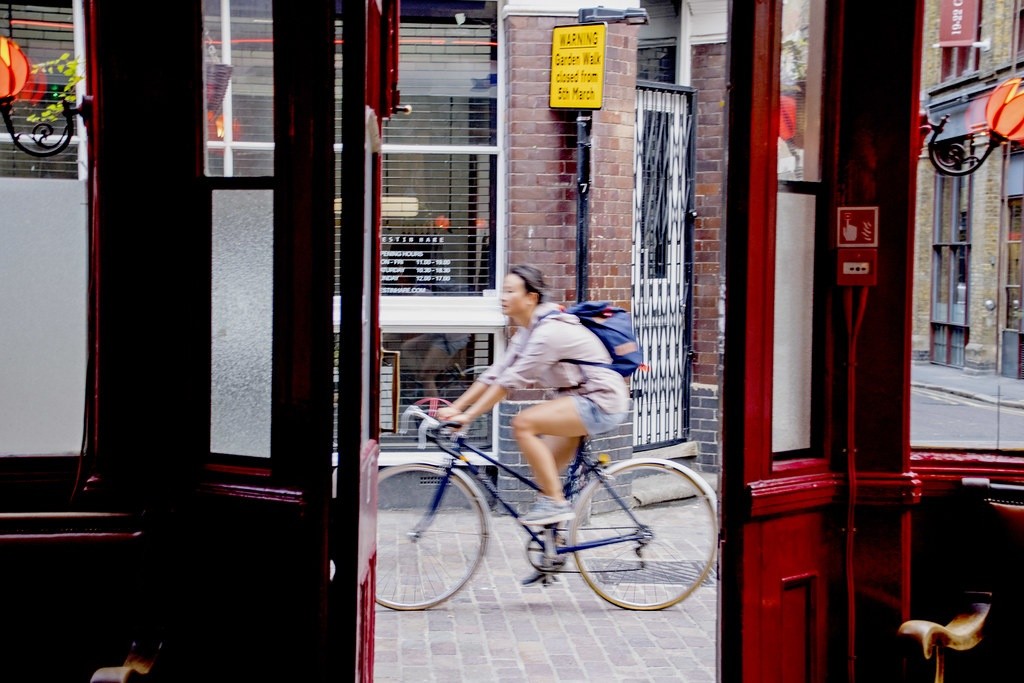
[383,351,493,449]
[374,404,720,611]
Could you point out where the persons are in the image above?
[431,263,631,588]
[394,234,471,407]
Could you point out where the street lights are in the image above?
[574,5,650,529]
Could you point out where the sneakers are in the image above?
[518,495,574,526]
[521,553,564,587]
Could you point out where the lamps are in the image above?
[0,36,94,157]
[919,77,1024,176]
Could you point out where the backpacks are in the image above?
[531,300,641,376]
[419,273,469,297]
